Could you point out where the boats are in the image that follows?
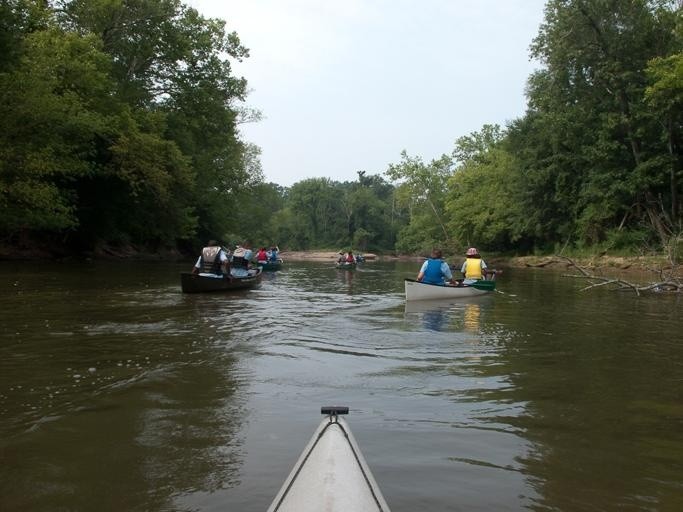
[404,270,503,300]
[181,266,263,293]
[336,262,356,269]
[257,262,282,271]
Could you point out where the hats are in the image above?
[466,248,478,255]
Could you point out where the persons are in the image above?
[187,237,278,279]
[415,248,459,287]
[461,247,488,281]
[336,249,362,265]
